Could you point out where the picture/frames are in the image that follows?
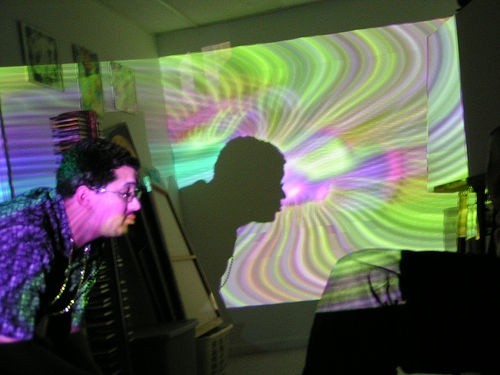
[71,44,105,117]
[20,23,65,91]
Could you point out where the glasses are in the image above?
[92,189,141,200]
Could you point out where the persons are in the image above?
[0,136,148,375]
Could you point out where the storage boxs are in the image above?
[133,319,198,375]
[198,322,235,374]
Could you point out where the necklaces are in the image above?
[50,233,87,316]
